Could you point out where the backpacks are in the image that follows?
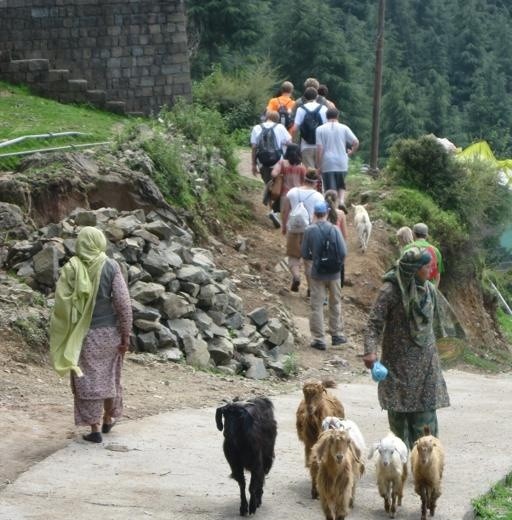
[300,103,322,144]
[418,245,438,280]
[256,121,281,166]
[313,223,337,273]
[278,97,291,129]
[288,186,318,235]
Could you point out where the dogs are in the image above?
[351,202,372,254]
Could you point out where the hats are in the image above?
[413,222,429,235]
[314,201,328,213]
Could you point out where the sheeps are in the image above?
[307,415,370,520]
[215,396,278,517]
[295,379,345,500]
[367,431,409,519]
[410,424,446,520]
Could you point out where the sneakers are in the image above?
[332,333,347,346]
[338,204,347,217]
[82,431,103,443]
[101,418,116,433]
[290,276,300,293]
[311,340,327,350]
[269,212,280,229]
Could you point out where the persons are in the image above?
[251,77,358,228]
[50,227,133,443]
[363,247,467,451]
[281,168,347,305]
[396,223,442,287]
[300,202,347,350]
